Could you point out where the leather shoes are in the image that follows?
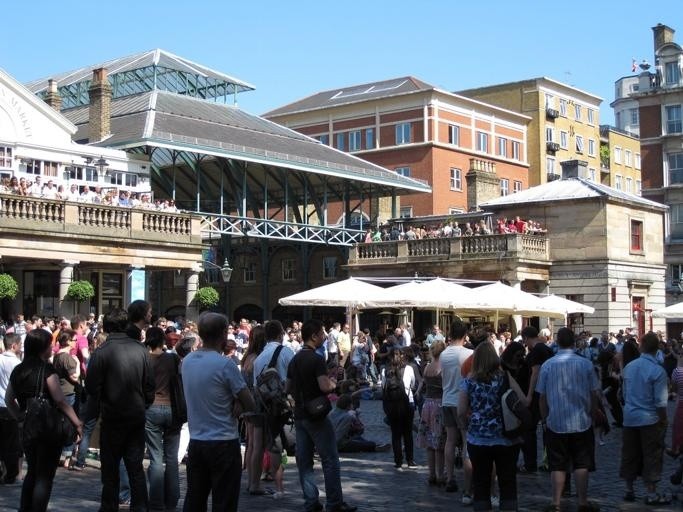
[246,471,357,512]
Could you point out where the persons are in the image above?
[0,300,683,512]
[363,215,549,244]
[0,172,181,213]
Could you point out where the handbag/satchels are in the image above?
[56,412,77,446]
[254,368,288,417]
[169,374,187,423]
[346,438,375,454]
[306,395,332,418]
[500,388,537,440]
[21,397,57,454]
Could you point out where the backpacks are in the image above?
[383,364,412,422]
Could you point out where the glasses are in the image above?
[71,339,77,342]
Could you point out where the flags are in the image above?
[632,59,638,71]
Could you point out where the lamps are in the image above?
[197,258,233,282]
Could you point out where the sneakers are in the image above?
[622,489,635,500]
[69,464,86,471]
[393,462,569,511]
[644,494,673,505]
[375,443,391,452]
[577,502,600,511]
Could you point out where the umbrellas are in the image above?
[278,275,596,331]
